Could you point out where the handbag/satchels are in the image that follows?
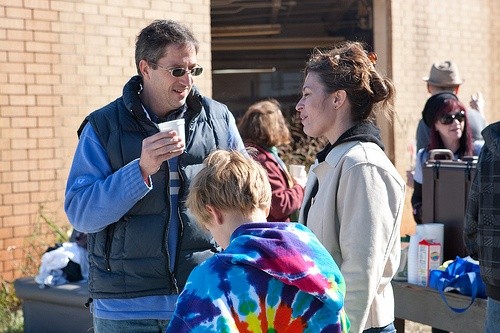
[428,256,489,313]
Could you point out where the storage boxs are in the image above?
[421,147,477,261]
[419,238,442,287]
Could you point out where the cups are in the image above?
[158,119,186,153]
[290,164,306,178]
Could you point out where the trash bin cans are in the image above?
[14,274,95,333]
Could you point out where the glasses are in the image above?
[434,110,466,125]
[138,57,204,78]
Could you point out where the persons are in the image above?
[294,40,406,333]
[64,20,249,333]
[237,100,305,223]
[461,121,500,333]
[406,62,487,189]
[163,149,351,333]
[411,92,482,225]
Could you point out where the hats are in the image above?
[421,92,458,131]
[422,60,463,86]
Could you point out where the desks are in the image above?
[392,279,488,333]
